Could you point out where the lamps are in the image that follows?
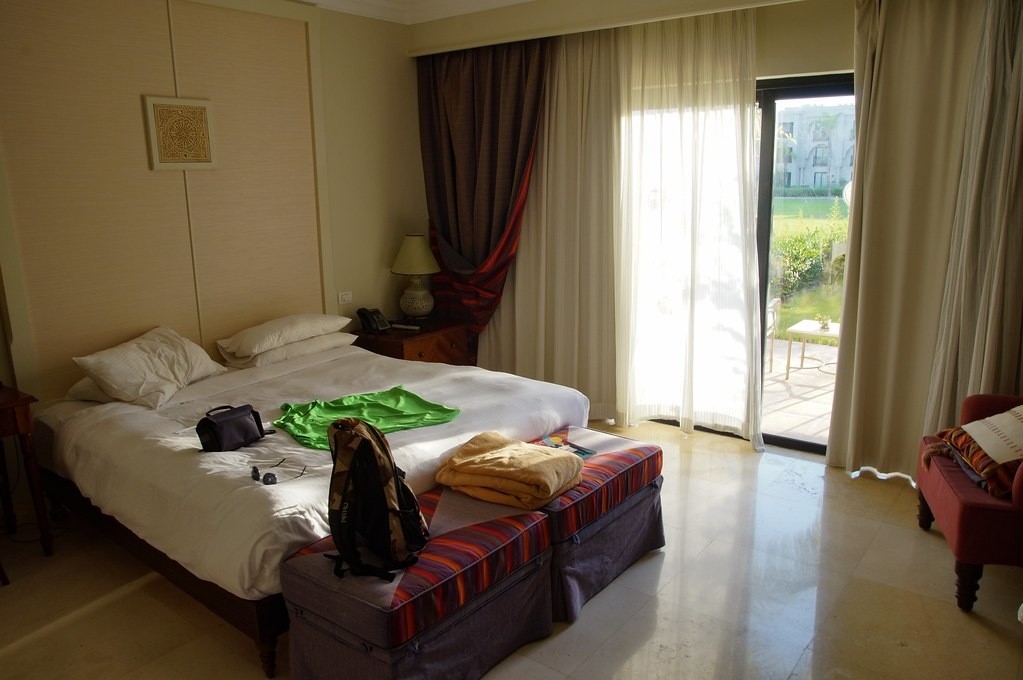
[391,232,443,317]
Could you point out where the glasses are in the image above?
[251,458,307,484]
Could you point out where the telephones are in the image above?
[356,308,392,333]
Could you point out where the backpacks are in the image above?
[323,417,432,583]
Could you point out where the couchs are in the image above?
[916,394,1023,611]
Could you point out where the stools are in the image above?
[280,487,554,679]
[527,426,665,626]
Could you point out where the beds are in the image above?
[28,343,590,680]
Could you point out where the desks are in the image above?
[785,320,840,381]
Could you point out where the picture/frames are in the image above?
[143,94,221,171]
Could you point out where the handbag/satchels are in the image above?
[196,404,263,451]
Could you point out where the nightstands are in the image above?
[352,319,472,364]
[0,382,56,585]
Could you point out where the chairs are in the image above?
[765,297,781,374]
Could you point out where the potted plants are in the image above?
[815,314,833,329]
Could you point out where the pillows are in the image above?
[934,405,1023,497]
[216,313,353,364]
[225,331,358,368]
[64,360,227,403]
[72,324,216,409]
